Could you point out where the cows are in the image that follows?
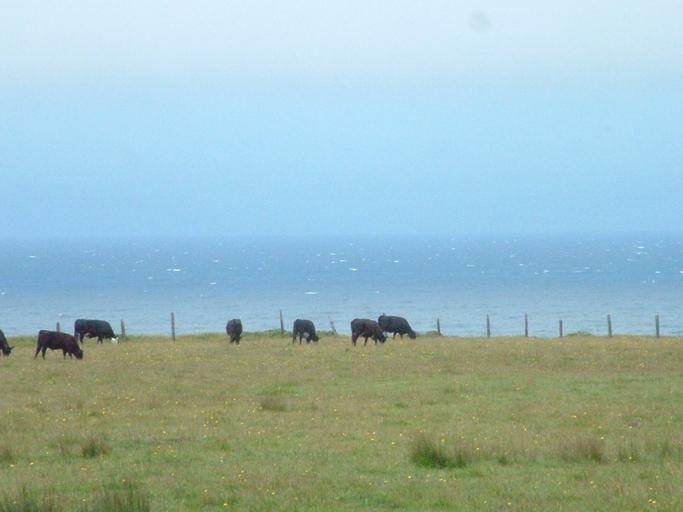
[351,318,388,346]
[226,318,242,344]
[291,319,322,344]
[74,319,120,344]
[0,329,15,357]
[378,315,416,339]
[34,330,84,360]
